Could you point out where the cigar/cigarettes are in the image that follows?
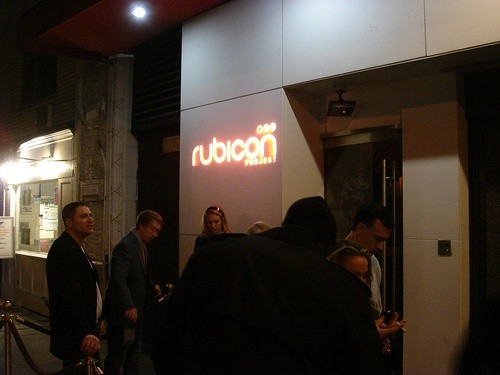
[396,322,407,333]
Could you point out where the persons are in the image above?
[345,203,394,316]
[46,203,103,375]
[194,207,228,252]
[101,210,163,375]
[150,198,385,375]
[325,240,407,353]
